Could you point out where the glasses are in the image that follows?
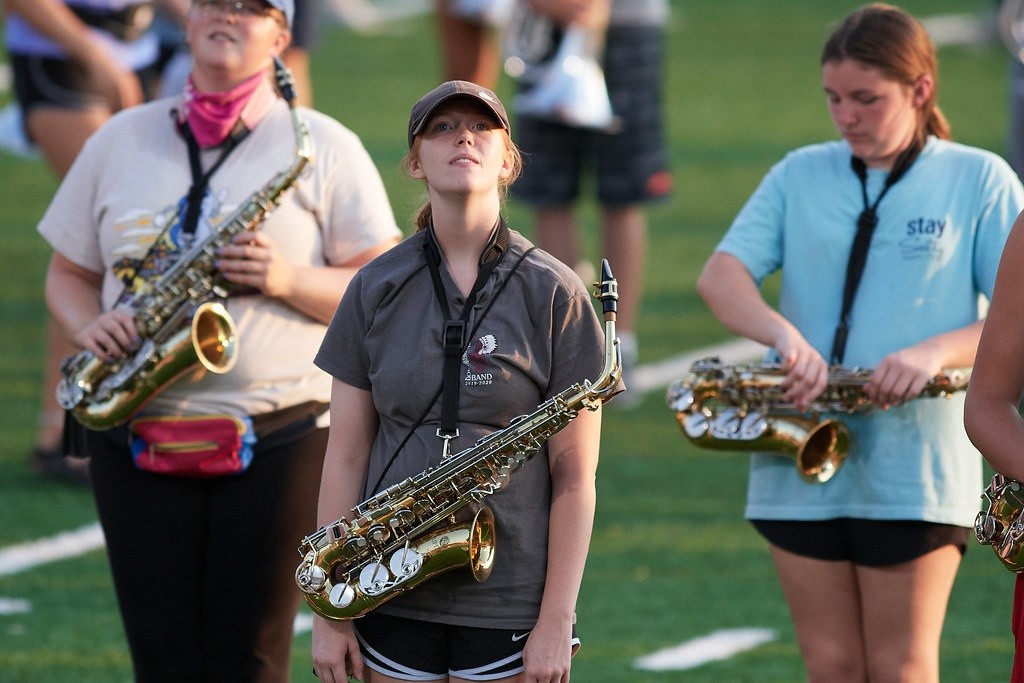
[190,0,284,28]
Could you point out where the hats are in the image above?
[267,0,295,29]
[407,80,510,150]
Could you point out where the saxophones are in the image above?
[666,357,976,486]
[974,473,1024,573]
[292,261,624,625]
[54,54,311,431]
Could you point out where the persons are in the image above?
[963,196,1024,677]
[2,1,192,476]
[272,0,321,103]
[308,83,627,683]
[696,1,1024,682]
[435,0,509,96]
[36,2,403,680]
[505,0,672,410]
